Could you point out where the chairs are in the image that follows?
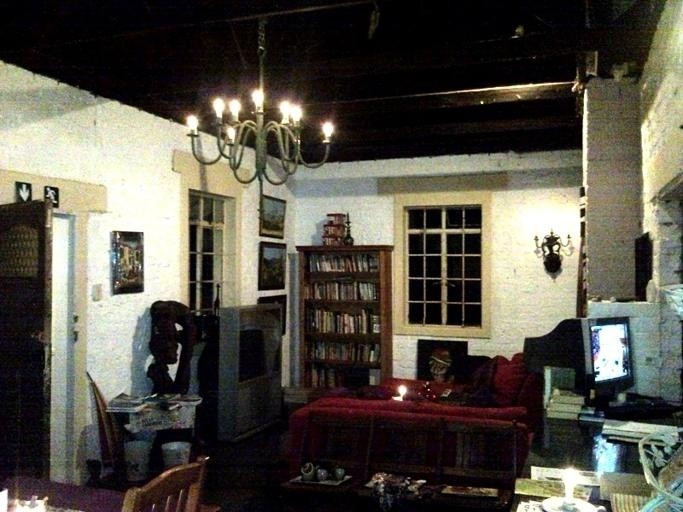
[120,455,209,512]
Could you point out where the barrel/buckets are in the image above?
[161,441,192,472]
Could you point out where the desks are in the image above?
[0,477,221,512]
[280,472,514,512]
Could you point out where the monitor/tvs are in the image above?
[522,315,635,407]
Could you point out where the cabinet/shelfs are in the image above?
[296,243,395,385]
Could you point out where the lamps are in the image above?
[186,0,336,190]
[534,229,572,273]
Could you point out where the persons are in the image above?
[45,187,58,204]
[18,183,30,201]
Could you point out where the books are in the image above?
[601,420,681,442]
[512,442,683,512]
[104,391,204,413]
[539,364,612,423]
[605,433,679,449]
[303,251,381,387]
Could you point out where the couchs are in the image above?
[287,352,545,490]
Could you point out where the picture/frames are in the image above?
[111,231,146,294]
[259,193,286,238]
[258,241,286,289]
[259,295,287,336]
